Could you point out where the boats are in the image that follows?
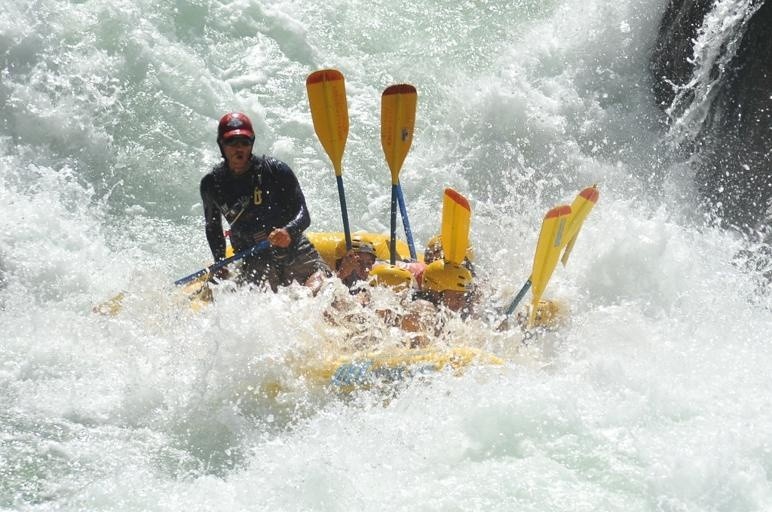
[177,229,509,416]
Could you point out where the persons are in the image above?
[200,111,343,312]
[335,235,475,349]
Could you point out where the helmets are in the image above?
[421,231,477,296]
[519,298,560,325]
[369,264,411,293]
[335,235,378,261]
[219,112,255,145]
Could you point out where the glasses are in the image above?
[224,137,251,146]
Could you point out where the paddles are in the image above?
[435,188,471,309]
[306,68,360,284]
[527,205,572,330]
[505,186,600,319]
[382,83,418,264]
[93,232,277,318]
